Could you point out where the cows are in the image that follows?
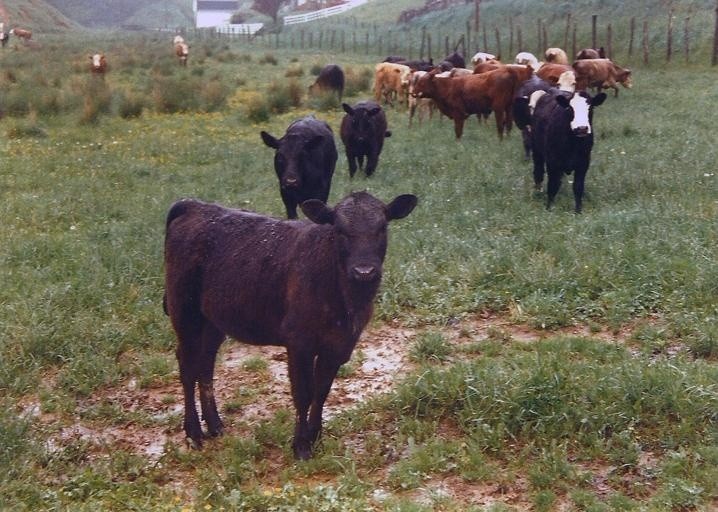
[260,114,338,219]
[89,53,107,74]
[340,100,393,178]
[162,192,418,461]
[308,65,344,103]
[371,46,634,142]
[175,43,191,68]
[532,87,607,214]
[0,23,43,50]
